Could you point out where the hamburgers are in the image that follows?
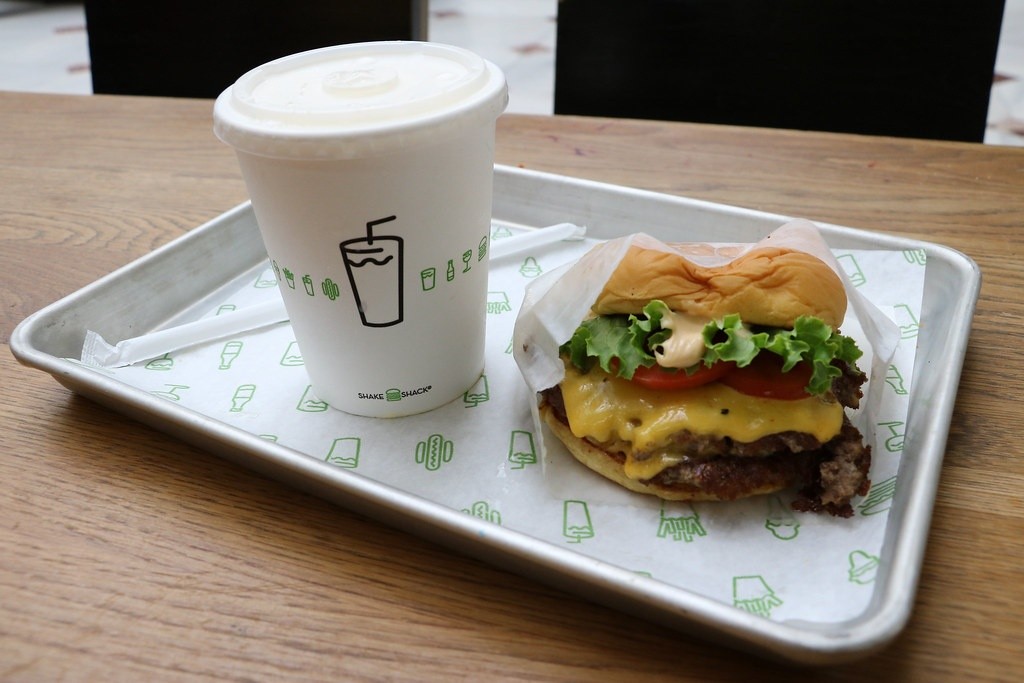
[535,242,875,516]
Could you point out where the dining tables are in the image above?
[0,89,1024,682]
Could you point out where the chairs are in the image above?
[554,0,1005,142]
[85,0,430,100]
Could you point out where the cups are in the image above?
[211,40,510,419]
